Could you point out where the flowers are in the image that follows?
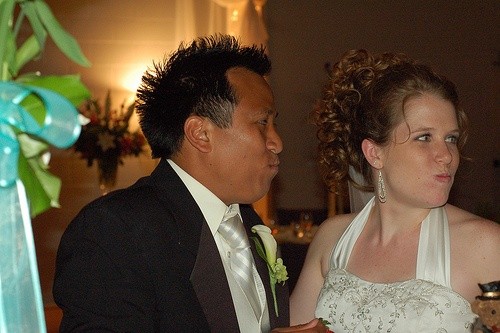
[75,90,148,188]
[251,224,289,318]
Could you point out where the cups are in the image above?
[290,212,313,238]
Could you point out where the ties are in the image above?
[217,213,269,332]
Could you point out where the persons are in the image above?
[53,34,334,333]
[289,48,500,333]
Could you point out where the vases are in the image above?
[98,156,119,199]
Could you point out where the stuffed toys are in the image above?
[470,281,500,333]
